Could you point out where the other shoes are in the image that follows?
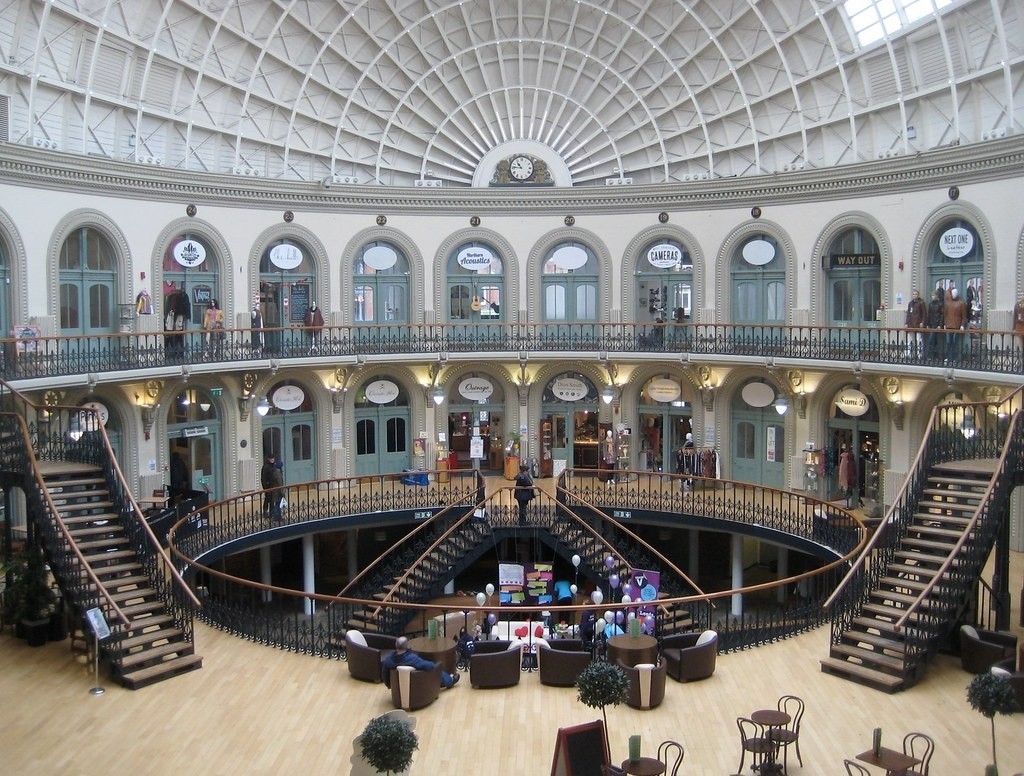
[447,673,460,688]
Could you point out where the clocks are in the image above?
[509,156,534,179]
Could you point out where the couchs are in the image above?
[660,630,718,683]
[840,516,898,557]
[469,640,524,689]
[345,630,398,684]
[988,658,1024,714]
[959,624,1017,675]
[813,509,858,555]
[616,656,667,710]
[492,621,549,646]
[389,661,445,712]
[535,638,591,687]
[129,508,177,556]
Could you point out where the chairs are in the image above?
[737,717,776,774]
[763,695,805,776]
[153,489,183,509]
[0,591,20,636]
[657,740,684,776]
[889,733,935,776]
[843,759,871,776]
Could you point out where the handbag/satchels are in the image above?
[532,485,539,496]
[211,322,227,340]
[280,497,287,509]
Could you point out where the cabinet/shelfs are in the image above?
[864,460,884,518]
[436,441,451,482]
[802,449,823,505]
[119,303,138,365]
[540,419,552,479]
[616,434,631,483]
[599,422,613,482]
[490,447,503,470]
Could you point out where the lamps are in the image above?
[69,417,83,442]
[599,374,614,404]
[432,372,446,404]
[772,389,791,415]
[960,408,976,439]
[256,390,273,416]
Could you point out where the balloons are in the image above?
[488,612,496,626]
[605,556,615,569]
[475,591,486,608]
[609,574,620,590]
[590,590,656,637]
[570,584,578,595]
[485,583,495,597]
[572,554,581,567]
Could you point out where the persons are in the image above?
[602,430,619,485]
[682,433,694,491]
[261,454,286,521]
[202,298,225,362]
[905,288,966,367]
[170,452,189,498]
[839,444,857,494]
[514,465,537,527]
[553,580,574,628]
[303,300,324,355]
[1011,291,1024,372]
[578,599,596,662]
[251,302,262,359]
[380,636,461,689]
[936,277,983,323]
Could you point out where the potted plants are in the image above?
[509,431,524,448]
[0,535,57,639]
[576,662,625,776]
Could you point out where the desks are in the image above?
[855,746,921,776]
[136,497,170,508]
[606,633,658,667]
[408,636,458,687]
[622,757,665,776]
[750,710,791,763]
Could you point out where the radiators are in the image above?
[792,308,810,353]
[332,311,343,350]
[702,308,716,352]
[425,310,436,348]
[427,442,436,471]
[237,312,255,354]
[884,469,909,508]
[334,448,346,479]
[791,456,806,491]
[30,315,57,370]
[239,458,255,492]
[519,440,528,466]
[885,309,905,358]
[609,309,622,350]
[140,472,163,511]
[518,309,527,349]
[136,314,158,362]
[987,310,1013,366]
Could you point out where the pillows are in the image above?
[535,626,543,637]
[515,626,528,637]
